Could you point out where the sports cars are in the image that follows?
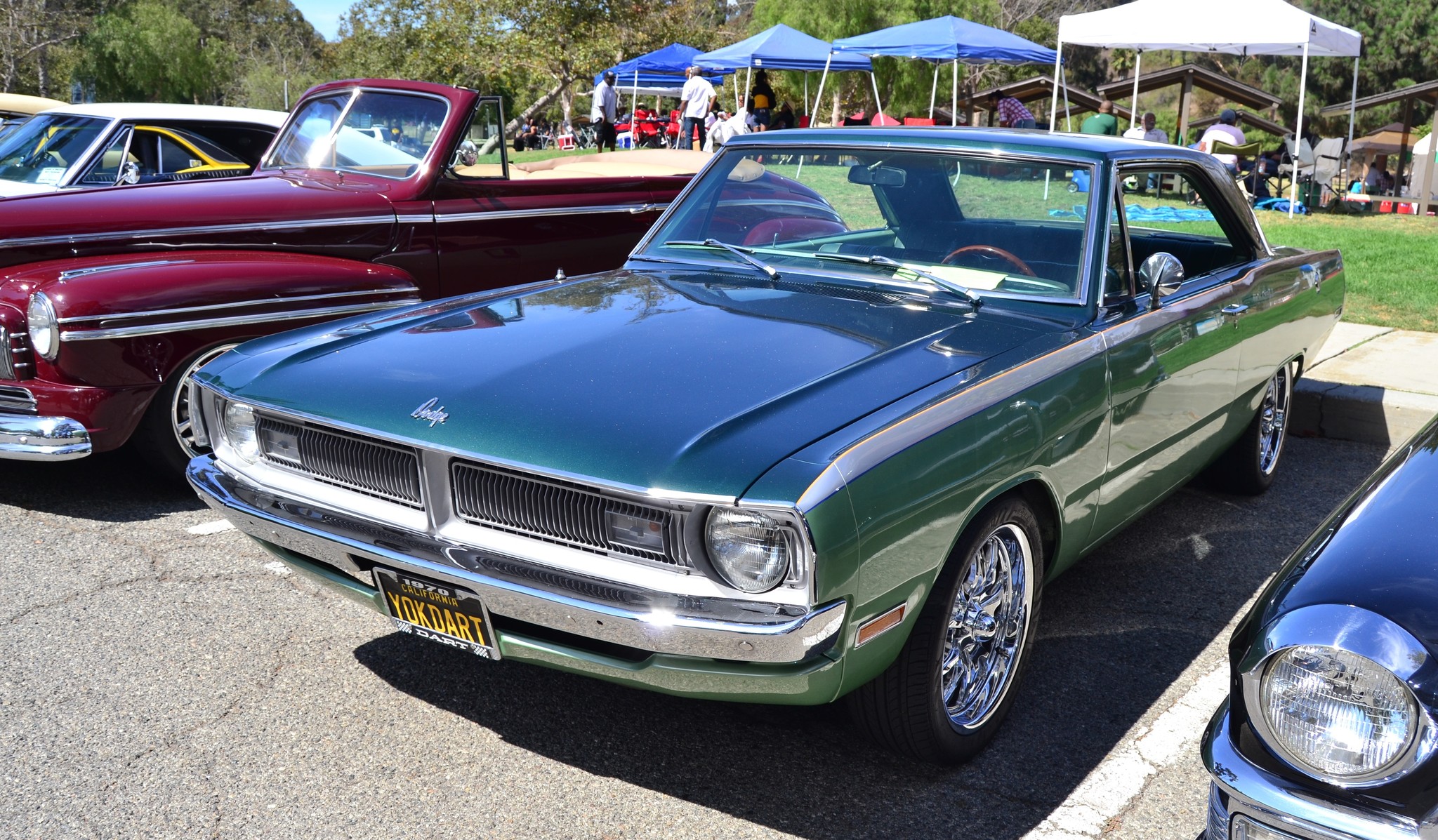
[0,80,851,495]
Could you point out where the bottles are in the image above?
[1258,156,1266,174]
[649,112,652,121]
[1385,189,1389,196]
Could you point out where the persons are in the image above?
[1321,178,1332,208]
[1347,174,1372,210]
[988,88,1036,179]
[1080,100,1118,136]
[752,71,777,164]
[592,71,616,153]
[518,116,553,151]
[1381,171,1394,195]
[676,65,720,151]
[768,99,796,130]
[617,102,651,146]
[1190,109,1246,205]
[1120,112,1169,193]
[717,94,756,132]
[1236,115,1311,209]
[1365,162,1381,195]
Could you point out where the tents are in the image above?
[676,23,884,149]
[796,14,1071,179]
[1410,132,1438,201]
[1340,122,1419,201]
[614,42,740,151]
[587,73,724,149]
[1043,0,1363,217]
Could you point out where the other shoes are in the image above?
[1136,186,1146,194]
[1004,170,1030,180]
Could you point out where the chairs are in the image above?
[1211,136,1351,210]
[531,106,710,149]
[769,112,1059,181]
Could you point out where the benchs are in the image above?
[896,219,1233,285]
[455,162,743,180]
[823,243,1125,296]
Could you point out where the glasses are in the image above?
[738,99,743,102]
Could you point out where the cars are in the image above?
[186,126,1347,763]
[1194,415,1438,840]
[0,91,422,196]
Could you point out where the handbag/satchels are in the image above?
[1285,137,1315,167]
[748,98,755,113]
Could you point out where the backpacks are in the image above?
[1328,197,1344,215]
[1342,200,1362,215]
[513,137,524,151]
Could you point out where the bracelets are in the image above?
[723,117,726,120]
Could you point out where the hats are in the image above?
[638,103,645,107]
[782,99,796,112]
[1221,109,1239,123]
[604,71,616,79]
